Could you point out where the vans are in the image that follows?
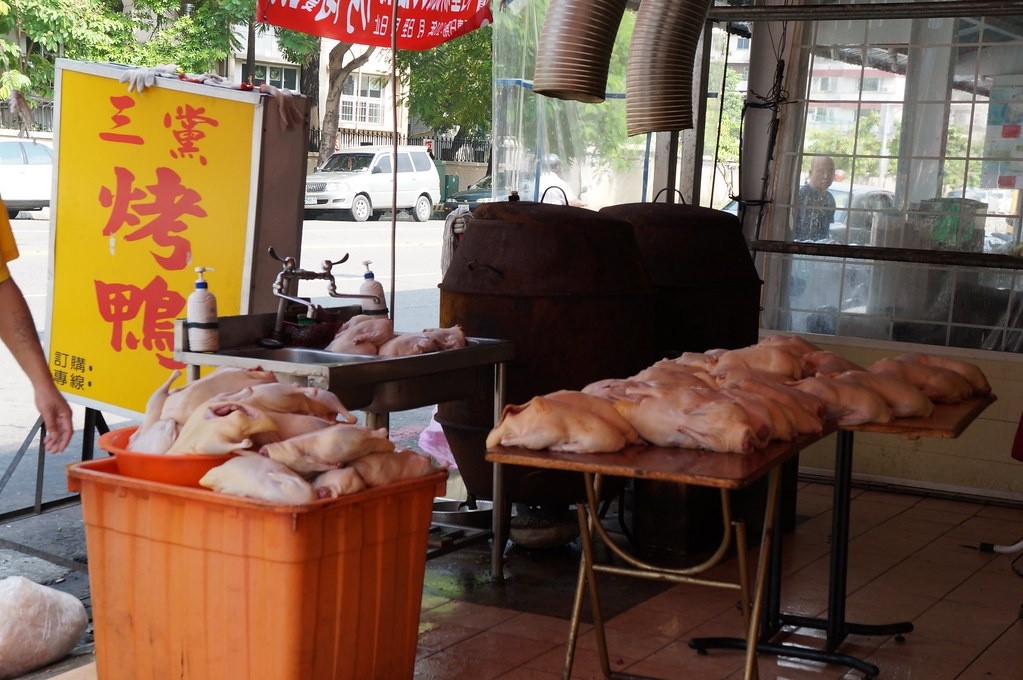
[0,136,54,219]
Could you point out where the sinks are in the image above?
[236,348,381,375]
[266,330,506,359]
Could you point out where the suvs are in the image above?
[304,145,441,222]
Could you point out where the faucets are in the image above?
[267,244,317,318]
[317,253,380,305]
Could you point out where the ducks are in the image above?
[485,334,989,453]
[324,315,393,356]
[378,323,466,356]
[125,363,451,504]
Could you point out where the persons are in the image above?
[0,193,75,454]
[535,152,587,207]
[793,156,837,242]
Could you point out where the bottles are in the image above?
[869,210,902,248]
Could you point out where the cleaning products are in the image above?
[187,267,219,352]
[359,260,389,318]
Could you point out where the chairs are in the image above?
[835,211,933,340]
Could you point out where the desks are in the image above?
[484,394,996,680]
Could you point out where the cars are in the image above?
[944,189,1013,214]
[720,181,895,242]
[444,169,574,216]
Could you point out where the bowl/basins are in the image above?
[96,424,238,491]
[431,499,493,530]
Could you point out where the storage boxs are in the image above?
[63,459,451,679]
